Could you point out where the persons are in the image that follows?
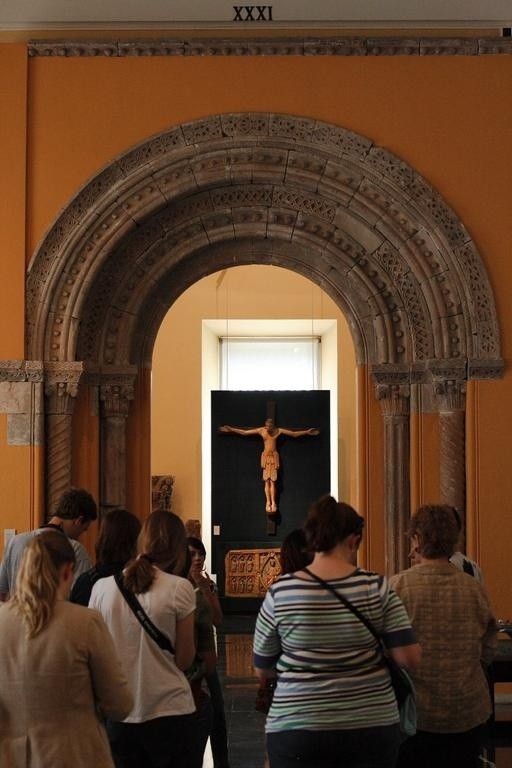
[443,503,484,584]
[88,508,197,767]
[220,415,320,514]
[252,494,420,766]
[0,530,135,767]
[1,489,98,602]
[71,509,141,607]
[186,538,228,768]
[278,529,314,574]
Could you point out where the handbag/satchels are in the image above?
[186,658,214,734]
[385,656,417,736]
